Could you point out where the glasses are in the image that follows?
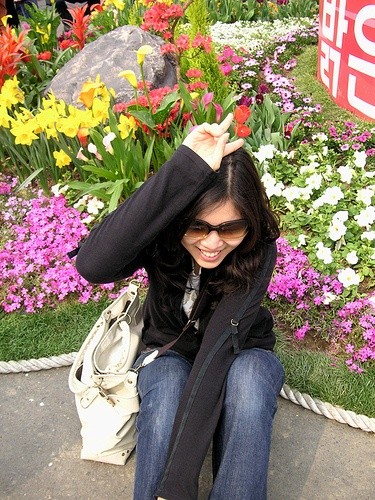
[184,219,250,240]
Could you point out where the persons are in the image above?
[76,112,286,500]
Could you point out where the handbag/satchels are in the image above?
[68,279,144,465]
